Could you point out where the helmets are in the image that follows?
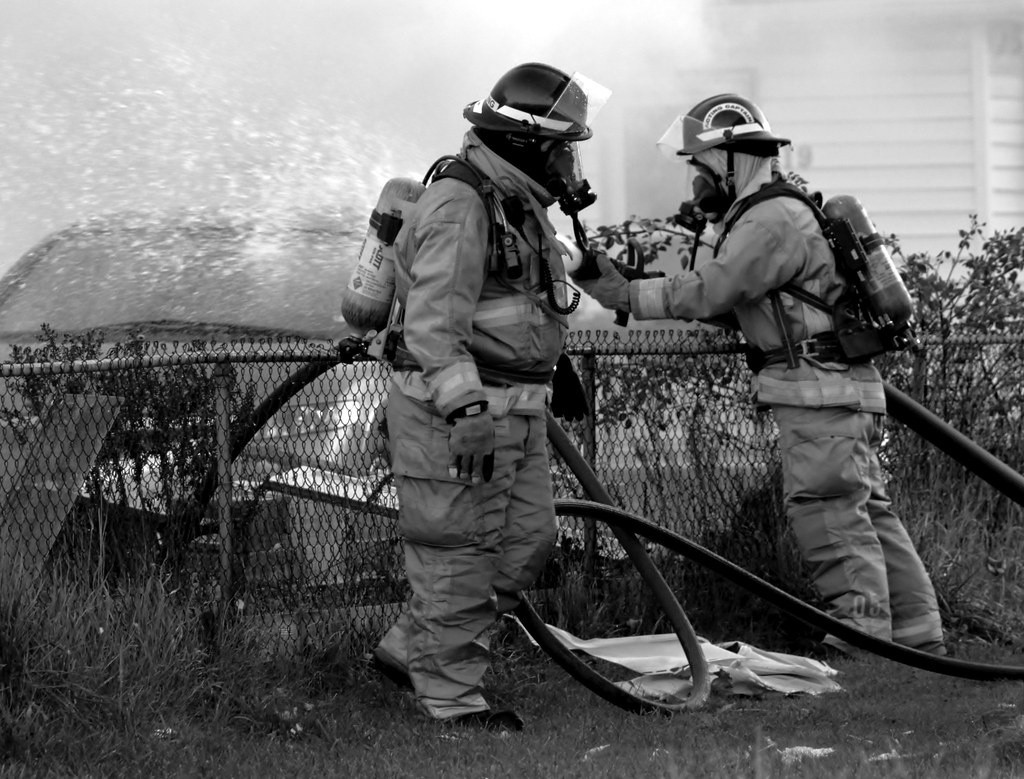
[676,93,792,155]
[463,63,593,141]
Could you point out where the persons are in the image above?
[573,93,949,660]
[372,63,592,730]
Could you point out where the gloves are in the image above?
[572,253,636,312]
[438,391,497,484]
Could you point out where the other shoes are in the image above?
[809,643,848,660]
[412,706,523,735]
[371,647,414,691]
[912,642,948,656]
